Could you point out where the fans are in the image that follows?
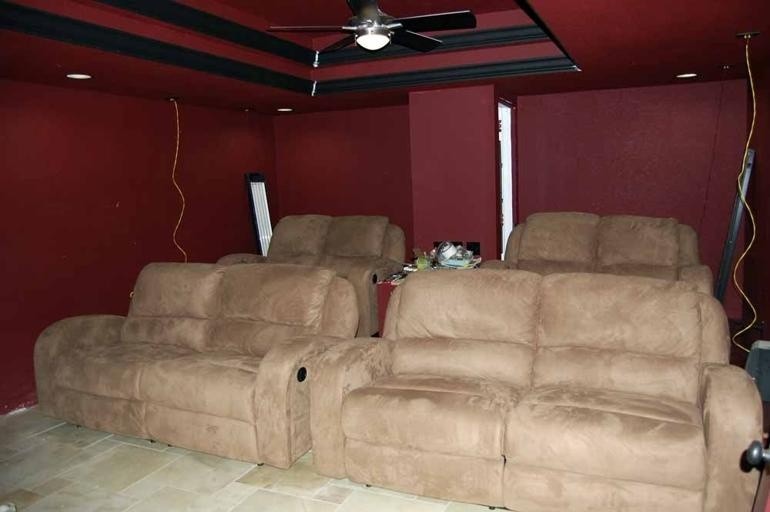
[264,1,476,54]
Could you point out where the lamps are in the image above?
[355,26,394,50]
